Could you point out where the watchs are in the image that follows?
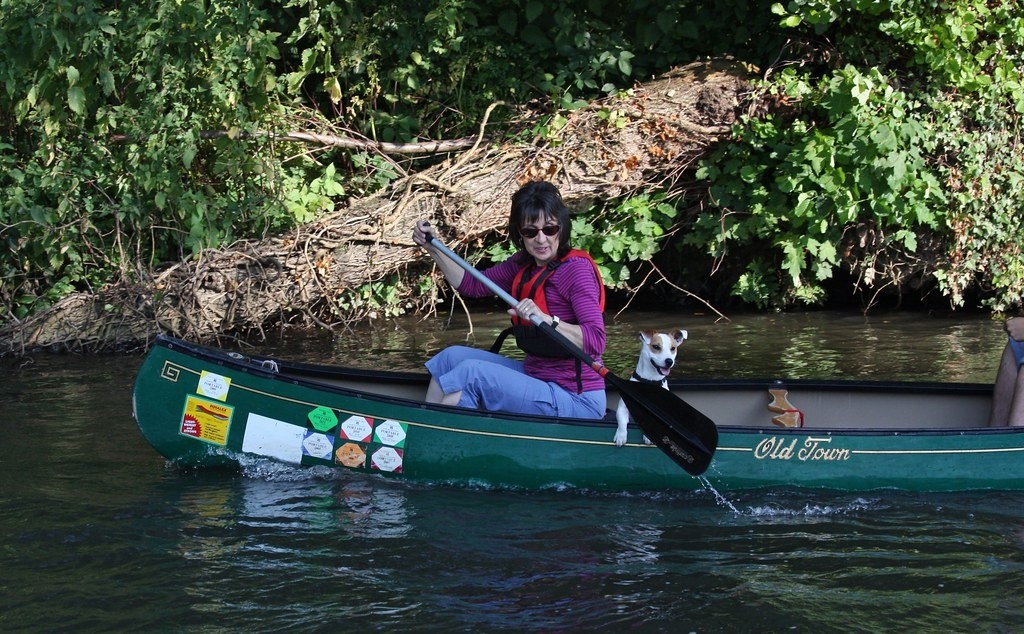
[551,315,560,329]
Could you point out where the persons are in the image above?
[411,180,607,420]
[988,307,1024,426]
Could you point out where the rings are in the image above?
[524,308,528,311]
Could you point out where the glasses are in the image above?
[519,225,561,239]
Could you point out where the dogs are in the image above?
[613,329,688,447]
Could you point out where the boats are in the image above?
[130,313,1024,497]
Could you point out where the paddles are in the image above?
[420,231,720,475]
